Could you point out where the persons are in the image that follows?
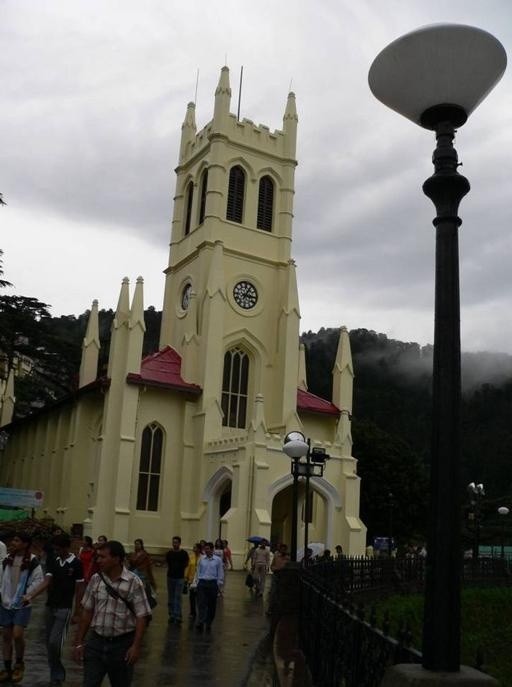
[364,540,426,562]
[0,527,159,687]
[161,535,292,633]
[302,545,347,563]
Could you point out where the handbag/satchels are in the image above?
[183,584,187,595]
[245,574,254,587]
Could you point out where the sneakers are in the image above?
[1,662,25,683]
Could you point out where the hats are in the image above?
[52,527,69,538]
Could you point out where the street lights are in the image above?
[282,431,309,561]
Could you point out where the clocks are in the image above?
[226,273,265,317]
[175,274,193,319]
